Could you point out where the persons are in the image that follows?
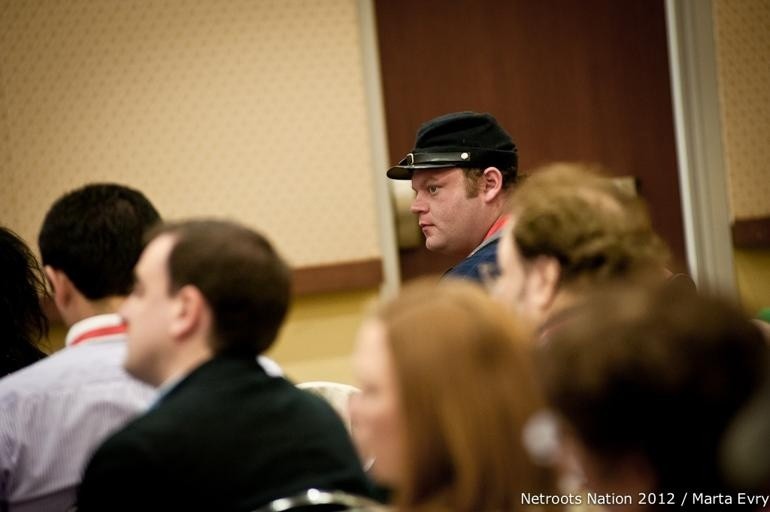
[493,159,673,346]
[0,224,51,378]
[72,212,371,511]
[343,268,573,512]
[0,179,286,504]
[528,272,769,511]
[385,106,516,295]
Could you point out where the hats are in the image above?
[388,116,517,178]
